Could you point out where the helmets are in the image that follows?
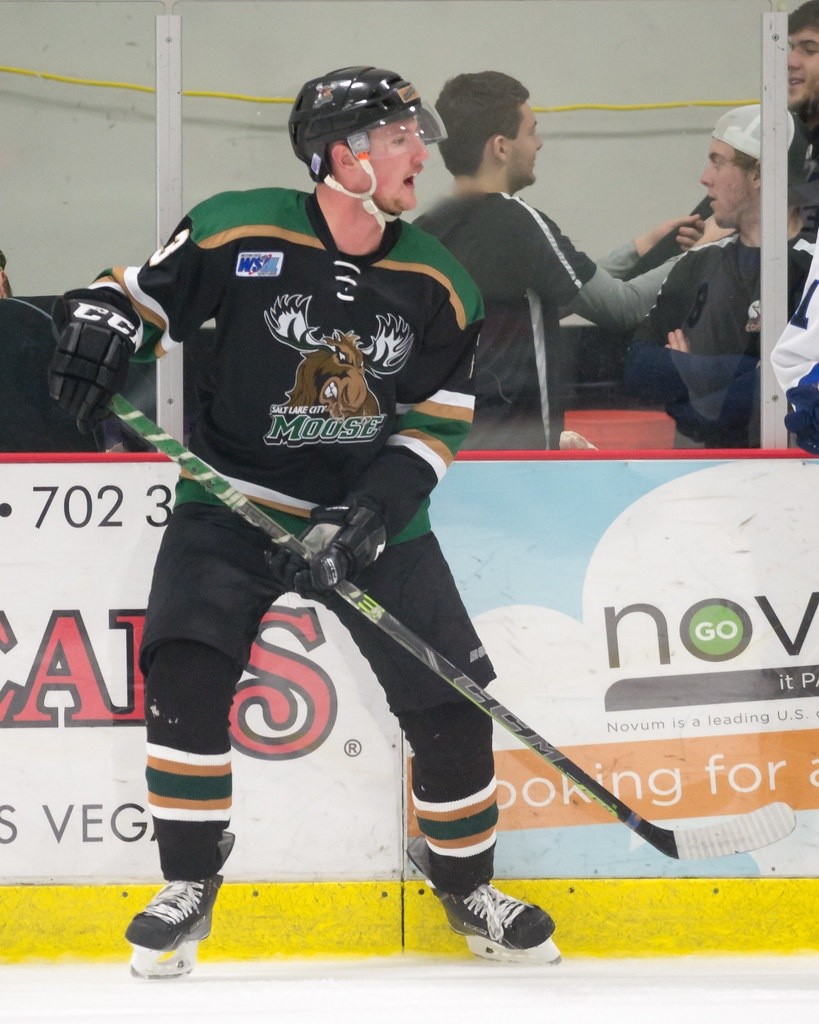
[286,66,449,183]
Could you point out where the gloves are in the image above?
[268,493,387,599]
[44,288,143,435]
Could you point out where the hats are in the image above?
[710,103,819,207]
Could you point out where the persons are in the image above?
[0,240,19,305]
[42,65,566,983]
[618,1,819,452]
[398,70,737,455]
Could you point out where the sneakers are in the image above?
[124,831,236,980]
[405,835,562,967]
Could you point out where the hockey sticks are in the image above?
[107,392,795,858]
